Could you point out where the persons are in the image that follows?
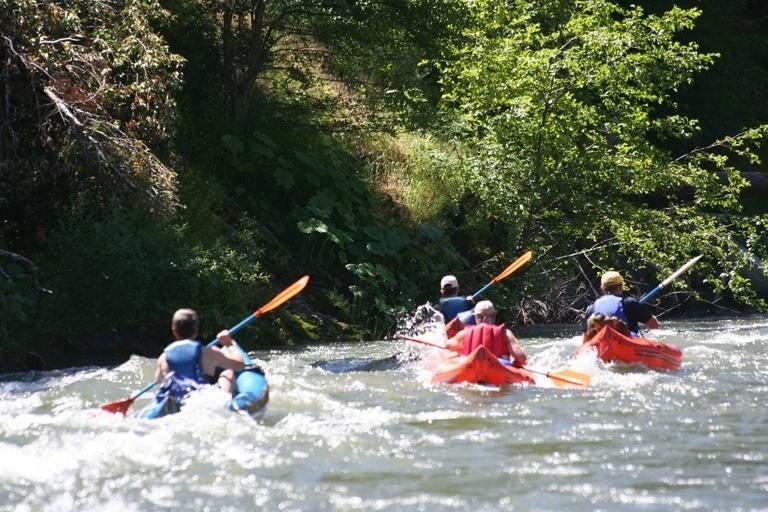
[156,309,244,413]
[417,275,475,335]
[446,301,527,369]
[582,271,660,347]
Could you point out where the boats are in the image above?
[124,341,270,422]
[571,325,684,372]
[429,346,537,386]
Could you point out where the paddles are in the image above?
[101,275,309,415]
[399,335,590,388]
[472,251,531,300]
[639,253,704,304]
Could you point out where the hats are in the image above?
[600,272,623,288]
[441,275,458,290]
[474,300,498,316]
